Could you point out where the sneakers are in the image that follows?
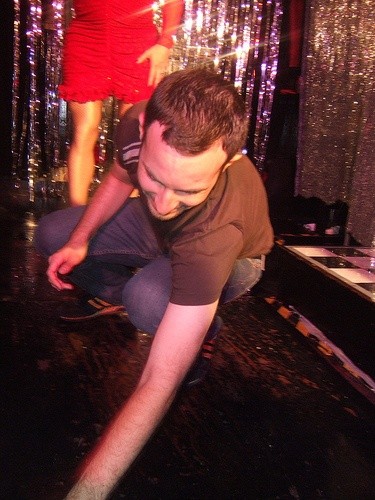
[55,291,125,321]
[180,315,224,394]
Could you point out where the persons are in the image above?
[57,0,188,204]
[29,63,280,499]
